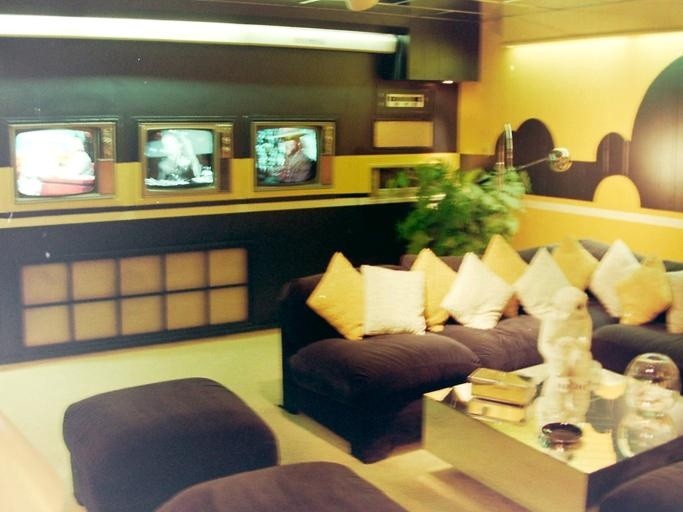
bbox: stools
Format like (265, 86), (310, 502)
(153, 461), (408, 512)
(63, 376), (281, 512)
(600, 460), (683, 512)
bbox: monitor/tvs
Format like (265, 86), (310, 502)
(7, 122), (116, 205)
(251, 121), (336, 192)
(138, 122), (233, 198)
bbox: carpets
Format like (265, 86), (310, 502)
(0, 328), (533, 512)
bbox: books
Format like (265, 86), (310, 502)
(466, 366), (545, 425)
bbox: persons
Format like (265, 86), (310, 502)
(277, 127), (314, 182)
(509, 117), (557, 195)
(151, 132), (202, 181)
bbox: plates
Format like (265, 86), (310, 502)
(540, 421), (584, 443)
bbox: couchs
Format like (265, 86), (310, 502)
(277, 237), (683, 464)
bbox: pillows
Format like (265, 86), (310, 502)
(552, 238), (600, 291)
(514, 248), (575, 323)
(614, 256), (672, 326)
(439, 252), (516, 330)
(481, 234), (530, 318)
(407, 247), (456, 332)
(589, 239), (641, 318)
(305, 252), (363, 342)
(666, 271), (683, 334)
(358, 263), (427, 336)
